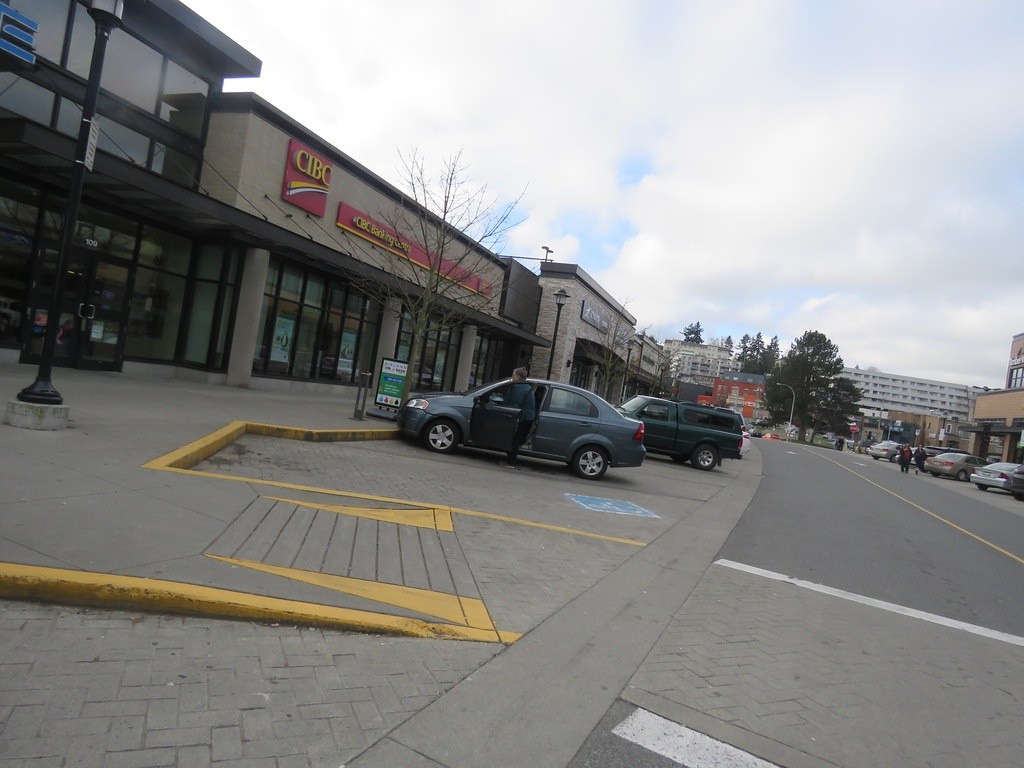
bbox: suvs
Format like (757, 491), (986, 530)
(924, 447), (967, 460)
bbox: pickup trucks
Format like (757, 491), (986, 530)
(613, 395), (744, 470)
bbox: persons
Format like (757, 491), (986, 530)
(913, 445), (926, 475)
(499, 367), (538, 469)
(835, 436), (845, 451)
(897, 443), (913, 474)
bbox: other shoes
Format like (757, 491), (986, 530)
(915, 470), (918, 475)
(498, 460), (516, 469)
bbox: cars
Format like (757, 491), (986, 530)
(868, 441), (905, 463)
(924, 453), (991, 481)
(747, 414), (879, 462)
(714, 406), (750, 459)
(971, 463), (1024, 496)
(397, 377), (647, 481)
(895, 447), (923, 466)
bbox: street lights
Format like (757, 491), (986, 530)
(777, 382), (796, 442)
(17, 0), (124, 404)
(546, 289), (571, 380)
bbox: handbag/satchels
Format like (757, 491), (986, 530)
(914, 457), (916, 461)
(898, 456), (904, 465)
